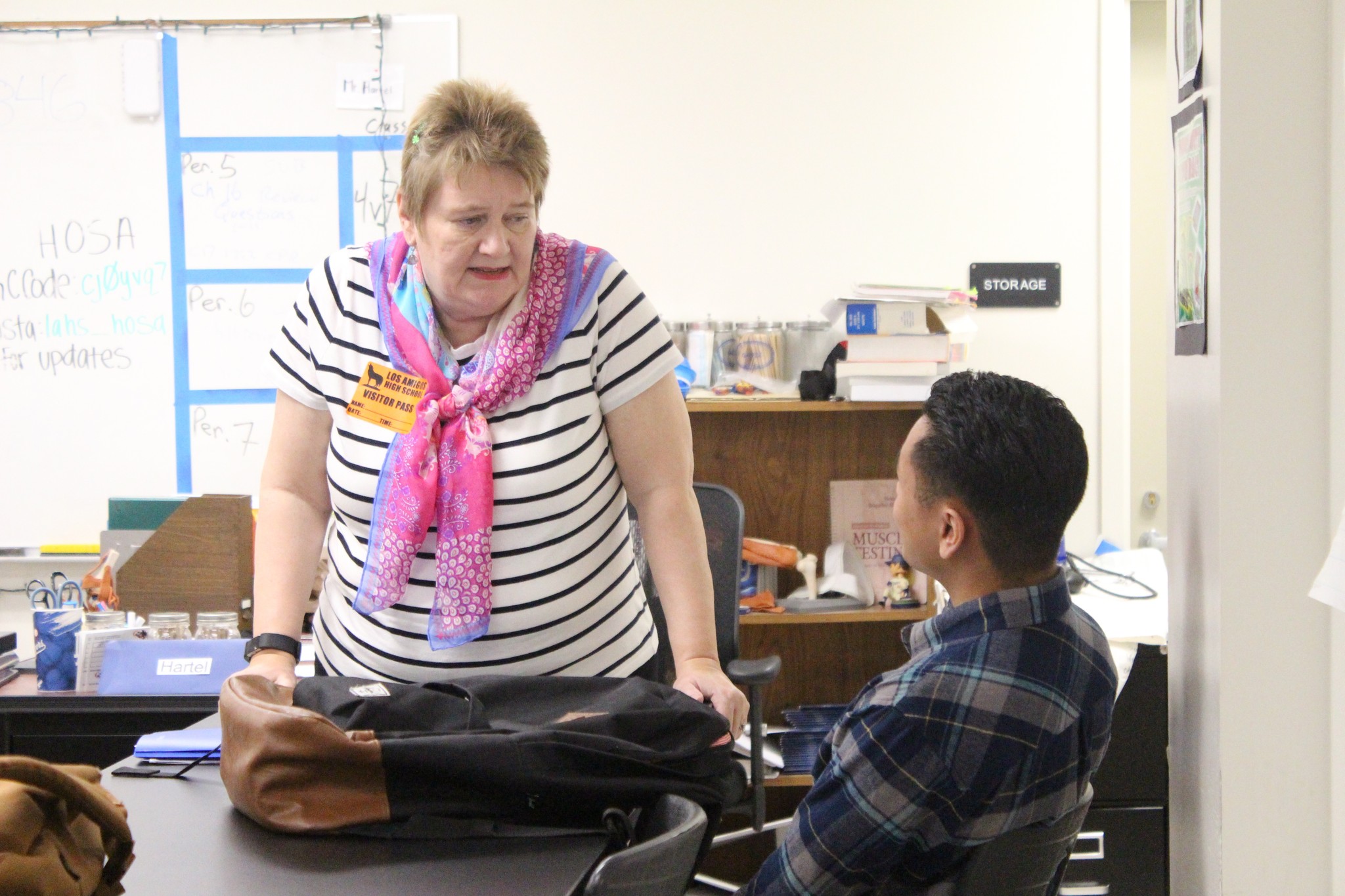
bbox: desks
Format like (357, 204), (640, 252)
(98, 713), (638, 896)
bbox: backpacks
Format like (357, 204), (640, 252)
(215, 670), (751, 836)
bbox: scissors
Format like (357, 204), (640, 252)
(26, 572), (72, 609)
(31, 581), (83, 610)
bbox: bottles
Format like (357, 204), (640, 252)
(662, 320), (831, 391)
(84, 611), (126, 631)
(195, 611), (241, 640)
(149, 613), (193, 641)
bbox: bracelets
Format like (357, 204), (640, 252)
(244, 632), (302, 664)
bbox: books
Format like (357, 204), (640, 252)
(0, 631), (20, 687)
(835, 285), (977, 402)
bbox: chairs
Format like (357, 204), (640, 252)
(957, 782), (1093, 896)
(584, 482), (790, 896)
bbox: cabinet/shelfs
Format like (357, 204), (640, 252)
(689, 402), (937, 624)
(0, 668), (219, 770)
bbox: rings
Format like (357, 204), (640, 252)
(739, 725), (745, 731)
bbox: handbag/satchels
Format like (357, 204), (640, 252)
(0, 759), (136, 895)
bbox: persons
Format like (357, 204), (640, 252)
(881, 555), (912, 603)
(748, 369), (1118, 896)
(246, 81), (751, 743)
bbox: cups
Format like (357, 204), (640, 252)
(32, 607), (85, 692)
